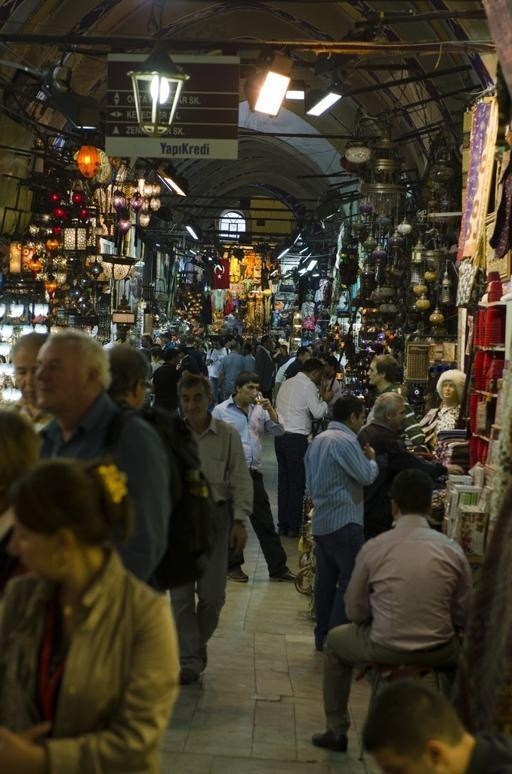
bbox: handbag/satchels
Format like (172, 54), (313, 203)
(140, 407), (218, 592)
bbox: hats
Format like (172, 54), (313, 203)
(435, 369), (468, 406)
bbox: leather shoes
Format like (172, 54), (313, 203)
(311, 731), (349, 753)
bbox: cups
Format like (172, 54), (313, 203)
(255, 393), (264, 405)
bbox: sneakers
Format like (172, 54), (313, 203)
(181, 670), (198, 685)
(314, 633), (331, 651)
(268, 568), (299, 582)
(277, 526), (287, 536)
(226, 565), (248, 581)
(286, 529), (301, 539)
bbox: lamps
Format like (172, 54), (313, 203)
(286, 79), (305, 101)
(244, 70), (289, 115)
(10, 146), (161, 300)
(345, 129), (454, 325)
(112, 295), (134, 343)
(305, 91), (342, 115)
(127, 34), (190, 137)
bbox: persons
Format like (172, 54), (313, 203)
(1, 457), (181, 774)
(303, 394), (379, 652)
(0, 409), (43, 599)
(357, 391), (465, 541)
(362, 676), (512, 773)
(312, 469), (473, 753)
(366, 354), (426, 445)
(103, 343), (202, 594)
(12, 332), (54, 434)
(273, 358), (334, 538)
(169, 374), (255, 684)
(34, 327), (172, 583)
(140, 316), (340, 412)
(418, 369), (467, 450)
(210, 370), (297, 582)
